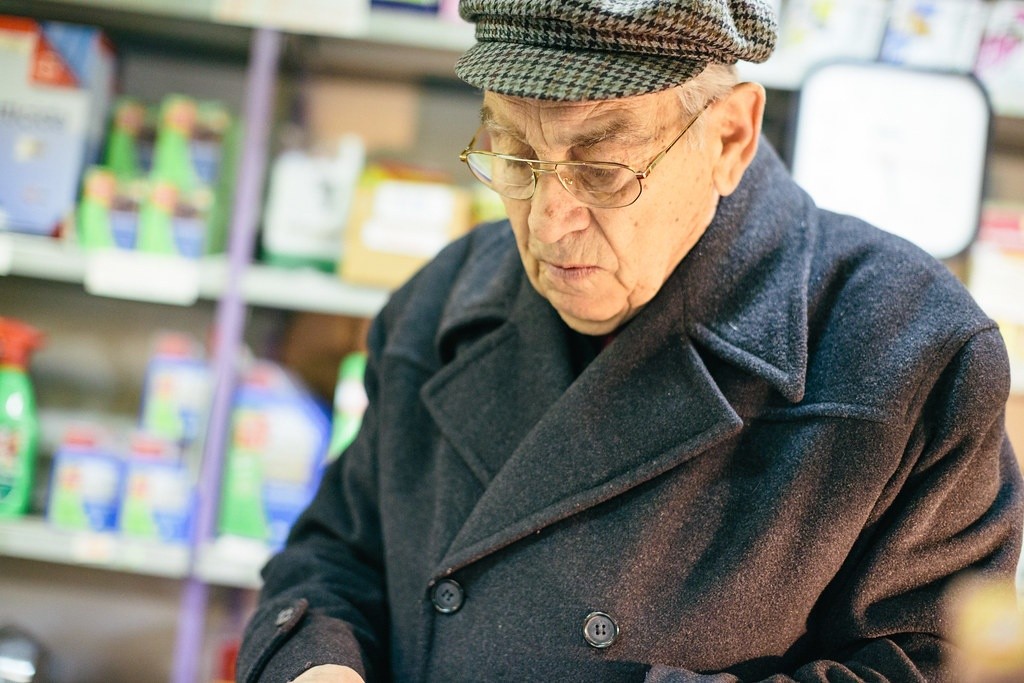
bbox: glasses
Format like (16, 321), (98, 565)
(458, 94), (714, 209)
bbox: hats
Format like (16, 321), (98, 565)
(451, 0), (778, 101)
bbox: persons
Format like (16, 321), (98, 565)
(234, 0), (1024, 683)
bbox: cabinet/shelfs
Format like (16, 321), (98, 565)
(0, 0), (1024, 683)
